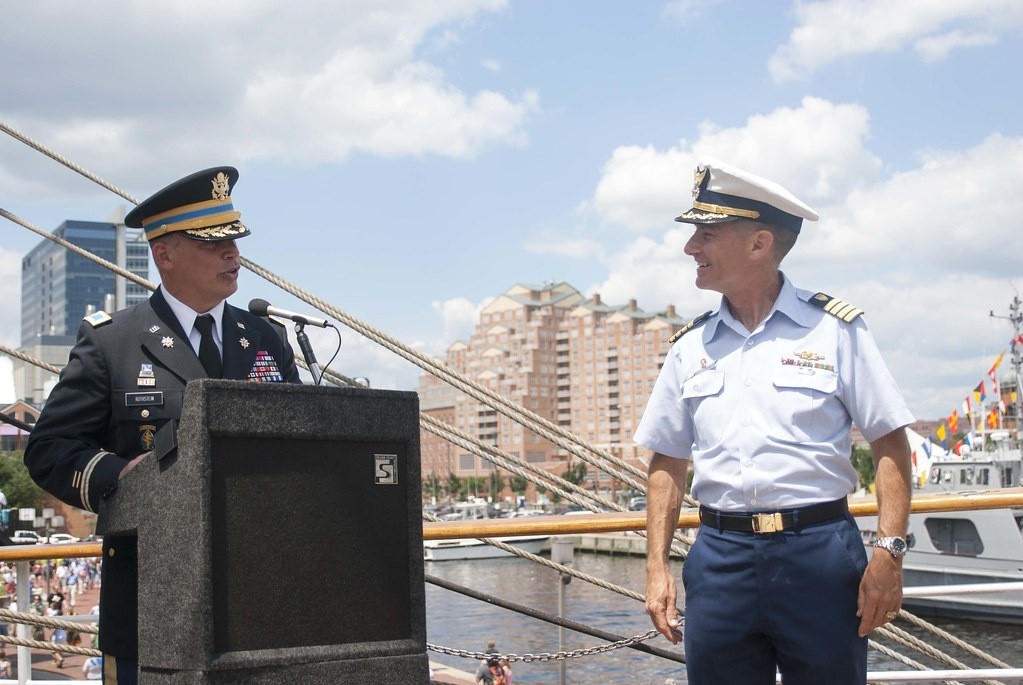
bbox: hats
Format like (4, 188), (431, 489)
(124, 166), (251, 241)
(673, 163), (818, 233)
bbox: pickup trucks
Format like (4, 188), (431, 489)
(8, 530), (47, 544)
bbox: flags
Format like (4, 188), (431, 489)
(975, 383), (985, 402)
(963, 398), (970, 414)
(953, 442), (963, 455)
(987, 331), (1023, 416)
(937, 425), (947, 450)
(922, 436), (932, 459)
(920, 471), (926, 486)
(948, 411), (958, 433)
(988, 410), (998, 428)
(964, 433), (976, 449)
(911, 450), (918, 466)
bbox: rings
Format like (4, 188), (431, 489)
(886, 611), (896, 619)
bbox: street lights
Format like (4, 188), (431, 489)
(550, 537), (575, 685)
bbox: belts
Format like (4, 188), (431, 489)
(700, 498), (850, 534)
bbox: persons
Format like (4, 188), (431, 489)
(475, 641), (512, 685)
(0, 556), (105, 680)
(633, 165), (916, 685)
(23, 166), (302, 685)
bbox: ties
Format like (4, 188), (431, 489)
(193, 315), (225, 381)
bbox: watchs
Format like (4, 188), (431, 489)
(873, 537), (907, 558)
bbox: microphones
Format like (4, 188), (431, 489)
(248, 299), (334, 329)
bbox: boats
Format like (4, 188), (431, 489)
(424, 536), (549, 561)
(846, 298), (1023, 624)
(438, 500), (503, 520)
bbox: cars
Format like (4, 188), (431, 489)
(37, 534), (81, 545)
(629, 498), (646, 511)
